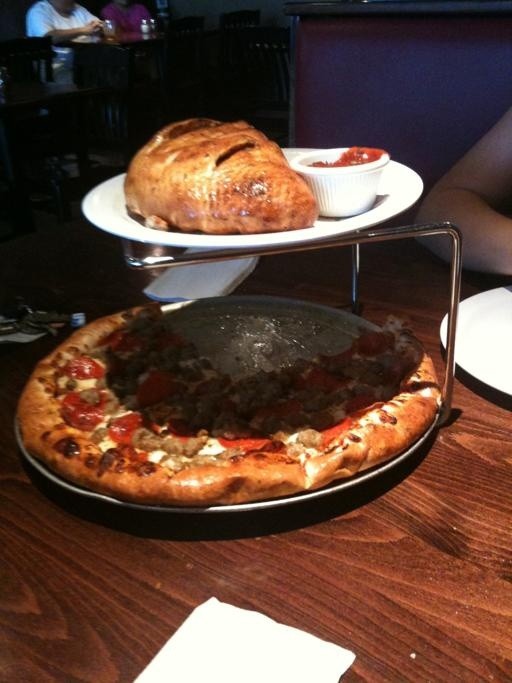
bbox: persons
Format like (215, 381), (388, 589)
(98, 0), (153, 33)
(24, 0), (105, 85)
(411, 104), (511, 281)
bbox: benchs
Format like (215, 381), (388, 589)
(286, 11), (511, 263)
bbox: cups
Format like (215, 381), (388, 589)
(288, 146), (390, 220)
(102, 19), (117, 39)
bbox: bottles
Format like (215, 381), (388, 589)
(140, 16), (155, 34)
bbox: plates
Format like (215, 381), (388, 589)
(438, 283), (512, 397)
(80, 149), (427, 252)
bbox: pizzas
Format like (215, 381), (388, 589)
(16, 301), (442, 507)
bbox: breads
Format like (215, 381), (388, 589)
(124, 116), (320, 235)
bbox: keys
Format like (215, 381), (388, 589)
(0, 301), (68, 340)
